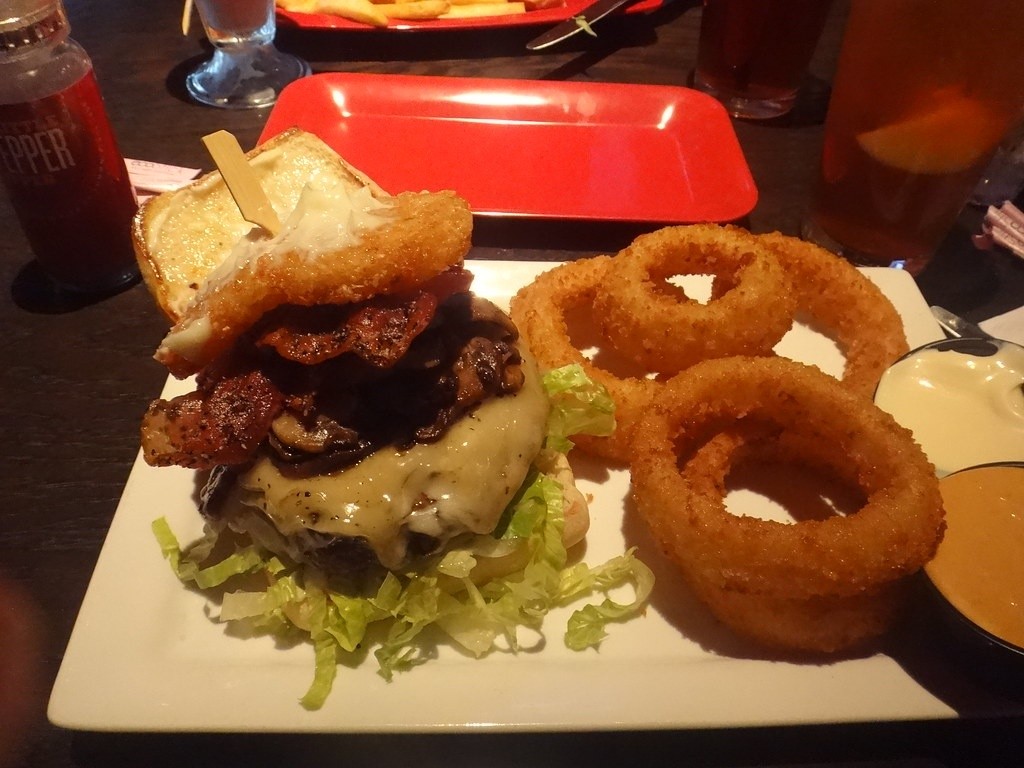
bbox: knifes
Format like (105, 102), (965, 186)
(526, 0), (632, 51)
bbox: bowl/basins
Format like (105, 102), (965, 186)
(865, 336), (1022, 662)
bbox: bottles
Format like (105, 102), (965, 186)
(0, 0), (158, 290)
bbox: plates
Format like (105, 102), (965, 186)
(256, 71), (762, 232)
(43, 259), (1023, 731)
(278, 2), (664, 31)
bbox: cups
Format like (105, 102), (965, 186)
(184, 2), (312, 109)
